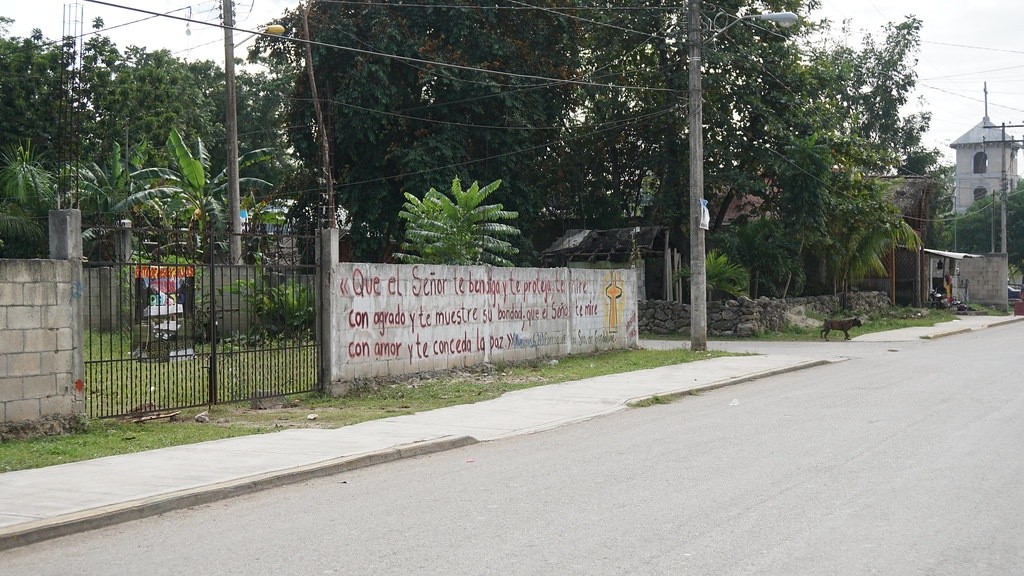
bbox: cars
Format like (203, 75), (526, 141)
(1008, 285), (1022, 299)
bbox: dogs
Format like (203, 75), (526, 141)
(820, 317), (863, 342)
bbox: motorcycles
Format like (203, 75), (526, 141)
(930, 288), (951, 309)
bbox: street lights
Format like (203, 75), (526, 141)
(688, 1), (797, 353)
(224, 1), (291, 265)
